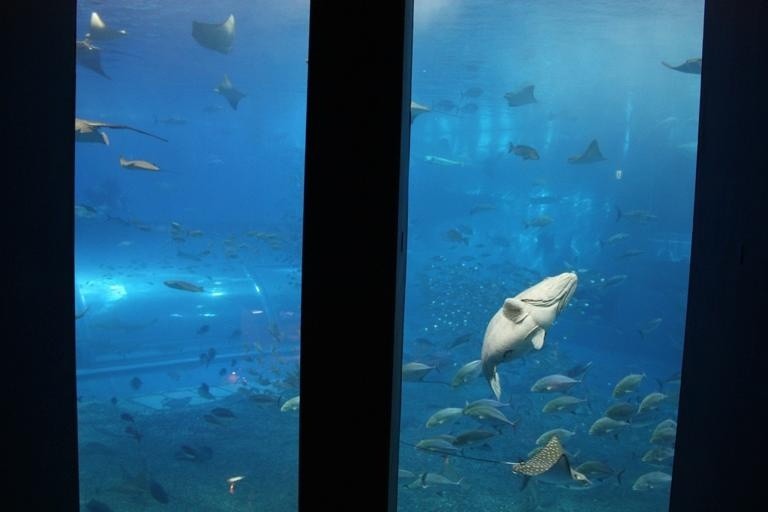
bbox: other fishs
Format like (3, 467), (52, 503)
(400, 197), (677, 496)
(72, 201), (303, 512)
